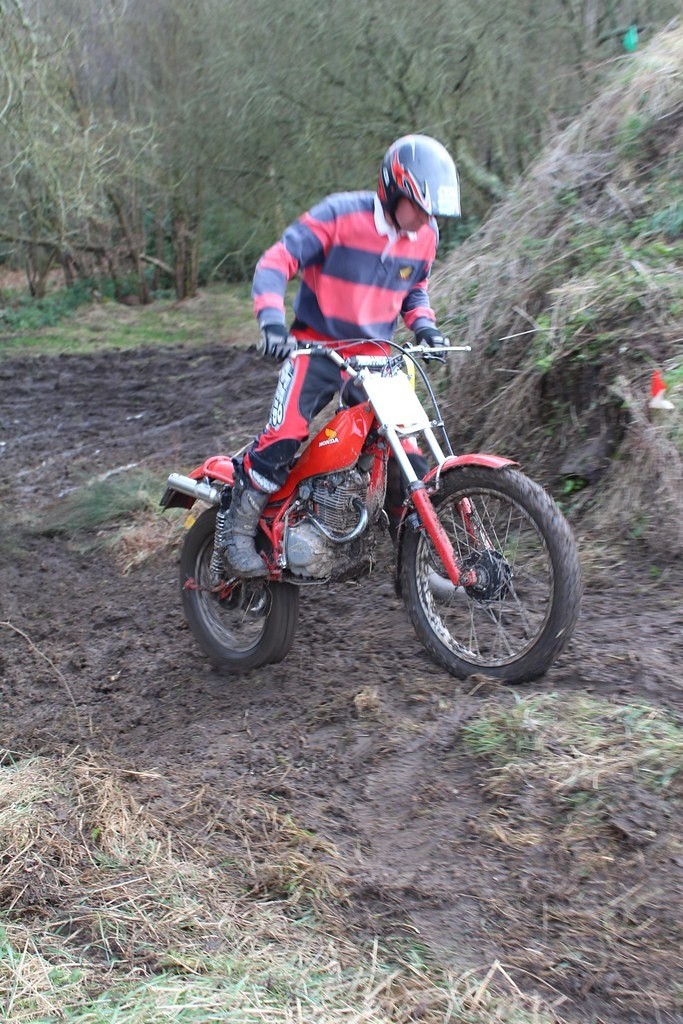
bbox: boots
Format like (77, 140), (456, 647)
(389, 519), (465, 601)
(218, 464), (271, 580)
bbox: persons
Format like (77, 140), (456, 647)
(221, 132), (464, 582)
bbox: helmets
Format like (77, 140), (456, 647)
(378, 134), (462, 216)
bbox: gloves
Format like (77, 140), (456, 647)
(416, 330), (452, 366)
(261, 325), (292, 362)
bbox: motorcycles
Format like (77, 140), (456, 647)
(159, 336), (581, 686)
